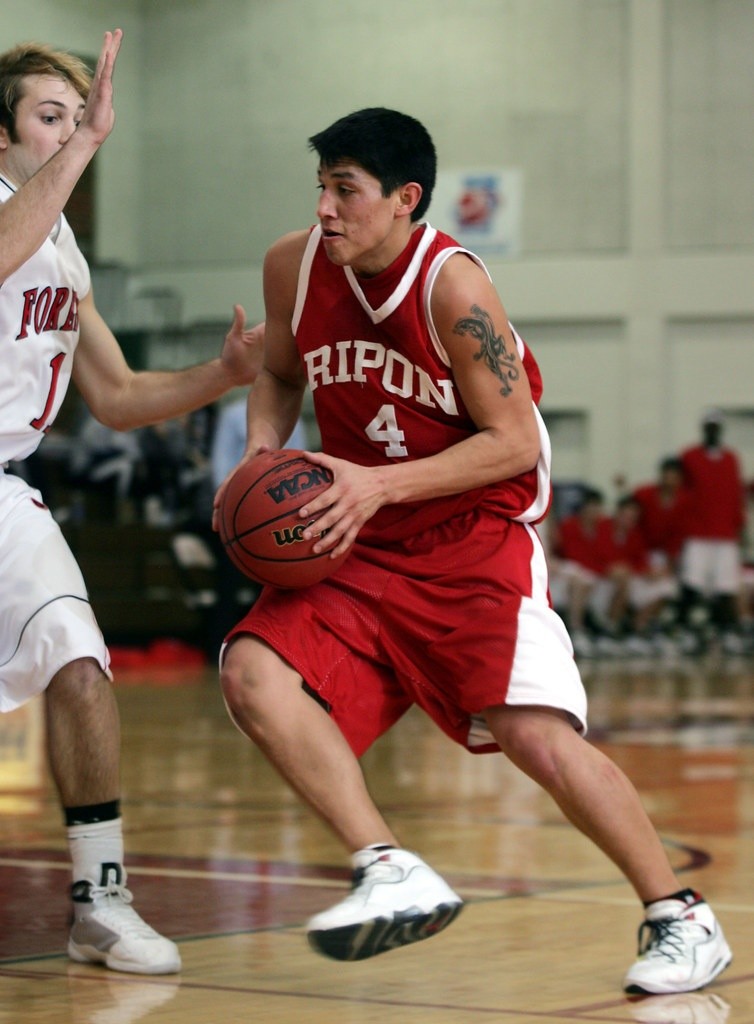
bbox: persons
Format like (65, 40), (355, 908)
(532, 506), (593, 659)
(0, 29), (266, 973)
(209, 397), (307, 669)
(563, 423), (748, 662)
(217, 106), (736, 994)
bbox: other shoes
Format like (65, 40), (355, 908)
(569, 629), (744, 658)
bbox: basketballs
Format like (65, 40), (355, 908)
(213, 447), (357, 595)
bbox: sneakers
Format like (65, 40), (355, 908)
(307, 849), (464, 962)
(622, 898), (733, 1003)
(66, 878), (183, 974)
